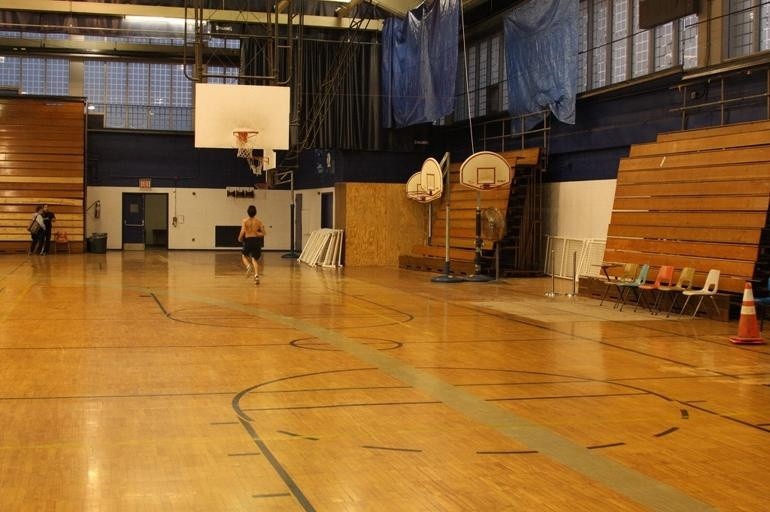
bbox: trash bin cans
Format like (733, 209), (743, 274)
(88, 232), (107, 253)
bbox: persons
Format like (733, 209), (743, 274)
(40, 204), (56, 255)
(28, 206), (46, 255)
(237, 205), (265, 285)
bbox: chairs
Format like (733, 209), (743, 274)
(600, 263), (640, 308)
(54, 231), (70, 255)
(680, 269), (722, 320)
(655, 267), (696, 317)
(592, 263), (626, 305)
(614, 264), (649, 312)
(634, 266), (673, 315)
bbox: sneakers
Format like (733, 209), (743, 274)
(245, 263), (260, 286)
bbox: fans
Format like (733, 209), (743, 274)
(481, 206), (509, 284)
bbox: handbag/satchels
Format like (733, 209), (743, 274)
(27, 216), (40, 235)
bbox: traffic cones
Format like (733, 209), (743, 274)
(728, 281), (770, 345)
